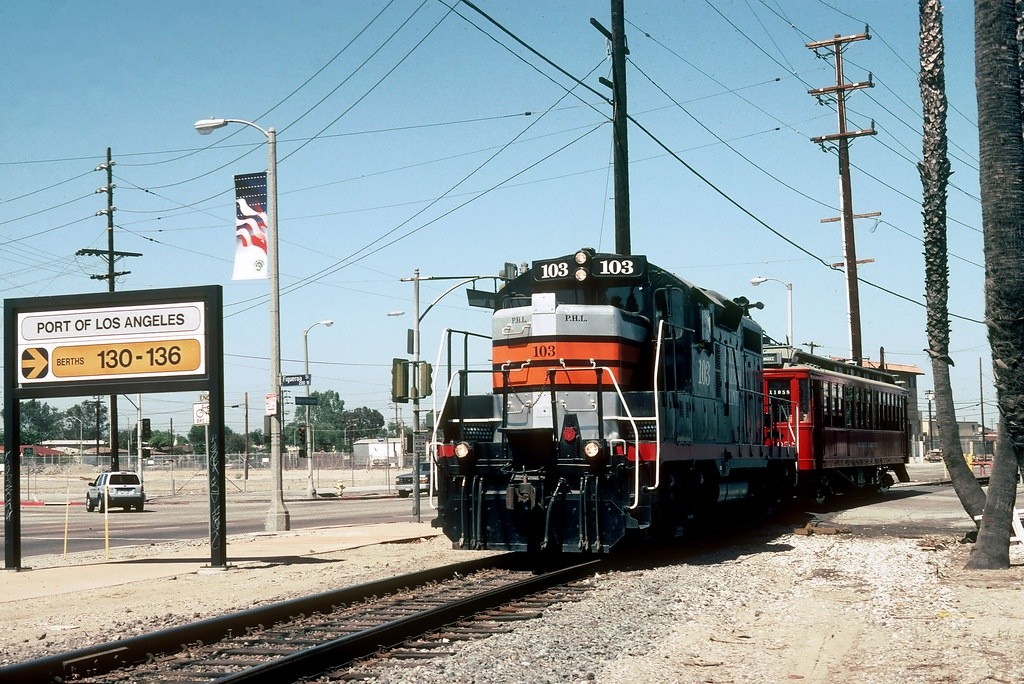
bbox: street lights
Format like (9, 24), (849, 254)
(750, 277), (796, 360)
(304, 320), (334, 500)
(194, 117), (294, 531)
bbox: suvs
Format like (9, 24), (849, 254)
(86, 469), (146, 515)
(395, 462), (439, 499)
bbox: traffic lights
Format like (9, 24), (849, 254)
(299, 428), (306, 446)
(391, 358), (409, 403)
(422, 363), (434, 396)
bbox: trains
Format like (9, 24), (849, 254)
(432, 246), (913, 570)
